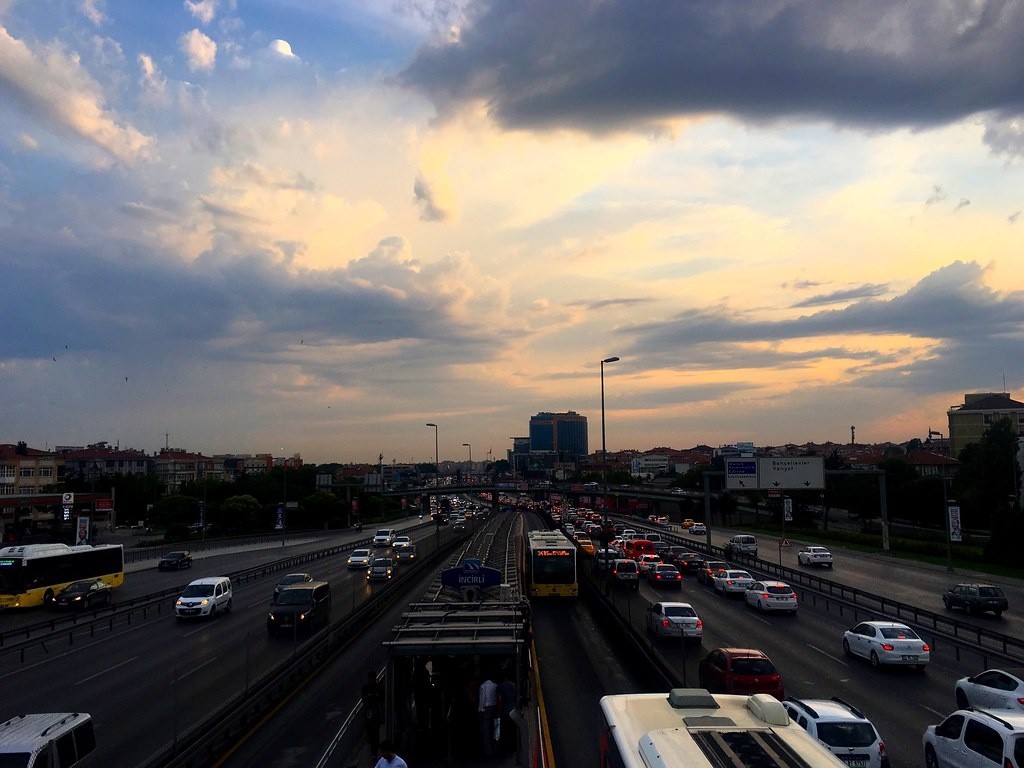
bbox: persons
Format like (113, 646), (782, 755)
(478, 669), (516, 756)
(373, 740), (408, 768)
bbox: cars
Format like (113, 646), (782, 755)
(273, 574), (315, 601)
(797, 545), (834, 569)
(158, 550), (193, 571)
(953, 667), (1024, 711)
(742, 579), (799, 616)
(645, 601), (704, 645)
(843, 620), (931, 673)
(428, 493), (758, 597)
(50, 580), (114, 611)
(697, 647), (786, 702)
(346, 528), (417, 586)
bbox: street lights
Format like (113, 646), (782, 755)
(461, 443), (474, 531)
(600, 356), (621, 555)
(425, 422), (440, 550)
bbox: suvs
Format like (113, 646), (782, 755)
(942, 582), (1009, 618)
(779, 695), (890, 768)
(922, 705), (1024, 768)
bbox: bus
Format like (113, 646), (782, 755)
(526, 528), (580, 601)
(594, 686), (852, 768)
(0, 544), (125, 609)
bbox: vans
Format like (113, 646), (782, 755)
(0, 710), (99, 768)
(267, 581), (332, 642)
(728, 535), (759, 557)
(173, 576), (233, 620)
